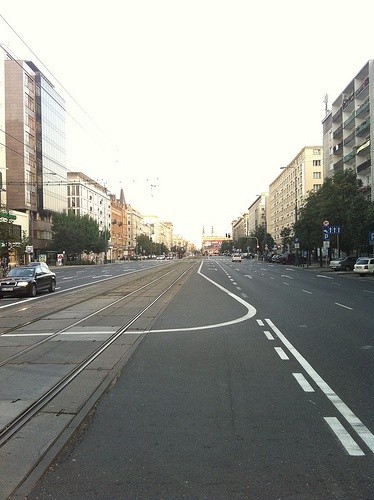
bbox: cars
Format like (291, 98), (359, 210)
(130, 254), (173, 260)
(0, 266), (57, 298)
(329, 256), (359, 271)
(270, 253), (308, 265)
(27, 262), (49, 269)
(232, 253), (242, 262)
(352, 258), (374, 276)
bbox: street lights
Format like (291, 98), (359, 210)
(280, 166), (297, 221)
